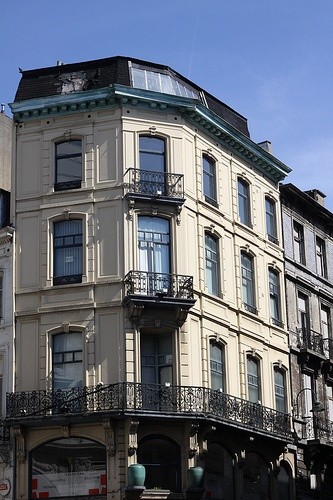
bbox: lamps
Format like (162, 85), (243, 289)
(295, 388), (326, 419)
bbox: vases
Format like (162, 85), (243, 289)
(127, 463), (146, 486)
(187, 467), (205, 490)
